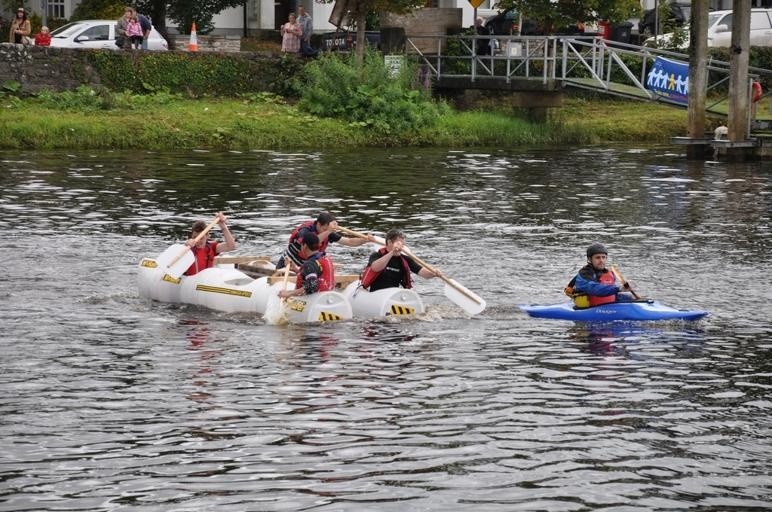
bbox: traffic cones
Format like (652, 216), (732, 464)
(184, 20), (201, 53)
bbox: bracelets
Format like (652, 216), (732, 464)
(221, 227), (226, 231)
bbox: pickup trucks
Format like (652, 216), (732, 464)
(319, 27), (382, 56)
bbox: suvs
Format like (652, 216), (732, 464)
(635, 5), (772, 63)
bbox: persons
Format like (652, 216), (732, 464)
(281, 12), (303, 53)
(564, 243), (633, 309)
(185, 211), (235, 275)
(115, 7), (151, 49)
(8, 7), (31, 44)
(34, 26), (51, 46)
(276, 213), (375, 269)
(277, 234), (335, 298)
(361, 230), (442, 292)
(470, 17), (490, 55)
(296, 4), (313, 50)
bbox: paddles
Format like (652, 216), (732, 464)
(336, 226), (410, 255)
(155, 217), (220, 280)
(264, 262), (289, 324)
(400, 246), (487, 315)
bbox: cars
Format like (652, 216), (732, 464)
(637, 0), (719, 34)
(482, 2), (589, 60)
(27, 14), (171, 53)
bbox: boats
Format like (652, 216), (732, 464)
(516, 291), (709, 323)
(132, 251), (426, 327)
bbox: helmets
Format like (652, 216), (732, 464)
(587, 243), (607, 257)
